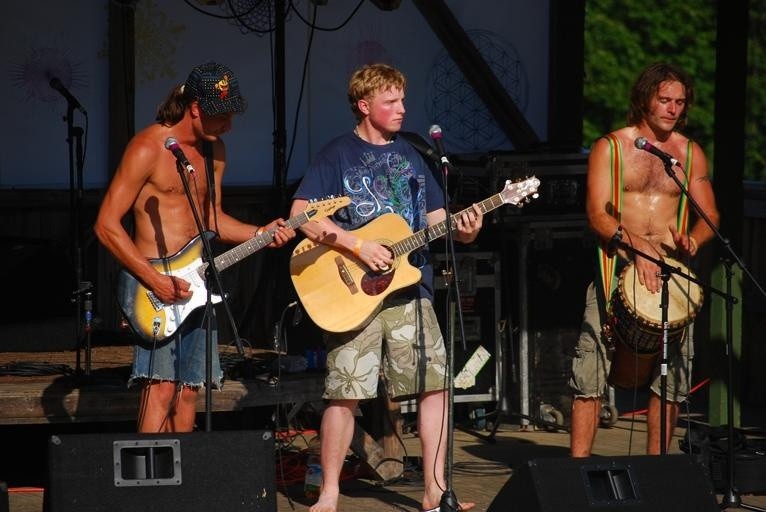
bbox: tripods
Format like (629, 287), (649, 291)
(629, 248), (766, 512)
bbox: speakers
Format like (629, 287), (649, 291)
(44, 429), (276, 512)
(485, 455), (721, 512)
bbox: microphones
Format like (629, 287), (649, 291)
(634, 136), (680, 167)
(165, 136), (194, 175)
(49, 77), (90, 117)
(606, 227), (623, 258)
(430, 124), (450, 168)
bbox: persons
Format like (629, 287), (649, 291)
(93, 61), (297, 433)
(567, 63), (720, 457)
(290, 63), (483, 512)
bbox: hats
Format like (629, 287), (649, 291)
(183, 61), (249, 117)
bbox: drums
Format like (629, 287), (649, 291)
(602, 256), (704, 390)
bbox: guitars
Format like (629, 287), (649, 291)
(289, 175), (541, 333)
(114, 194), (352, 349)
